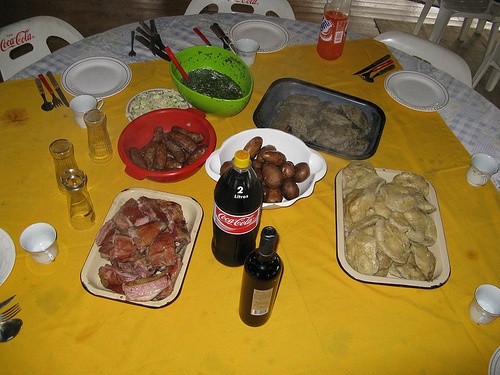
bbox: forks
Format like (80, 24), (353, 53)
(127, 30), (137, 57)
(0, 302), (22, 322)
(38, 74), (63, 109)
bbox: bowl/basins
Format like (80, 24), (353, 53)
(204, 127), (327, 209)
(117, 107), (217, 184)
(169, 45), (254, 118)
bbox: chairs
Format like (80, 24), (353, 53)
(184, 0), (296, 20)
(0, 16), (84, 81)
(373, 31), (473, 88)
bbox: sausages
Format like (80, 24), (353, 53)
(128, 125), (209, 172)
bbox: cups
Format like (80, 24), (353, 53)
(466, 153), (499, 188)
(469, 283), (500, 325)
(228, 38), (260, 68)
(19, 94), (115, 265)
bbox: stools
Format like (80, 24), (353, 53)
(413, 0), (500, 93)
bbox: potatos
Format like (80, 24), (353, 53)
(219, 137), (310, 202)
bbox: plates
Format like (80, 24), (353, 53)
(334, 169), (450, 290)
(61, 20), (449, 160)
(80, 187), (204, 309)
(0, 228), (16, 287)
(487, 346), (500, 375)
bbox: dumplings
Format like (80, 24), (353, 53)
(340, 160), (437, 282)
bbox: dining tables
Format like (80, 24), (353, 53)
(0, 13), (500, 375)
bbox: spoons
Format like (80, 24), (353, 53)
(0, 319), (23, 342)
(366, 64), (396, 83)
(35, 78), (54, 111)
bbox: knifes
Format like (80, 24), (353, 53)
(0, 295), (17, 308)
(135, 35), (171, 62)
(353, 54), (390, 75)
(46, 71), (70, 108)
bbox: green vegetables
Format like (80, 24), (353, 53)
(181, 69), (244, 101)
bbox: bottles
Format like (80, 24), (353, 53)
(237, 225), (284, 330)
(316, 0), (354, 60)
(211, 149), (263, 267)
